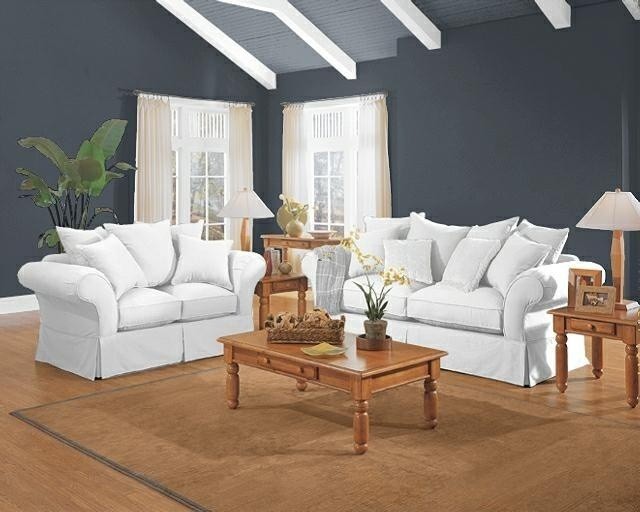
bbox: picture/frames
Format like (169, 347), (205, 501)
(567, 268), (603, 310)
(574, 286), (616, 314)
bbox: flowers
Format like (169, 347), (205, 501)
(284, 203), (308, 219)
(341, 238), (413, 320)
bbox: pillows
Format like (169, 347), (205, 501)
(348, 225), (402, 275)
(516, 219), (572, 266)
(79, 235), (144, 300)
(363, 211), (426, 241)
(466, 215), (520, 284)
(136, 214), (203, 265)
(484, 231), (552, 298)
(441, 239), (502, 293)
(405, 211), (470, 281)
(103, 218), (178, 288)
(55, 226), (104, 269)
(383, 239), (433, 286)
(171, 235), (234, 288)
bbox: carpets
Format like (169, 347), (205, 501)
(7, 363), (623, 512)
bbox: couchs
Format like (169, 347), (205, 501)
(17, 246), (267, 380)
(304, 246), (606, 387)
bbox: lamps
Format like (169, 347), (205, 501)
(216, 188), (274, 250)
(575, 188), (640, 308)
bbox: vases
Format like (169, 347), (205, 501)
(285, 220), (305, 238)
(356, 320), (391, 350)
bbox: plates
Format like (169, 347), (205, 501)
(307, 230), (338, 239)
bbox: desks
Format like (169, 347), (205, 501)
(251, 274), (307, 330)
(546, 307), (640, 407)
(259, 232), (341, 294)
